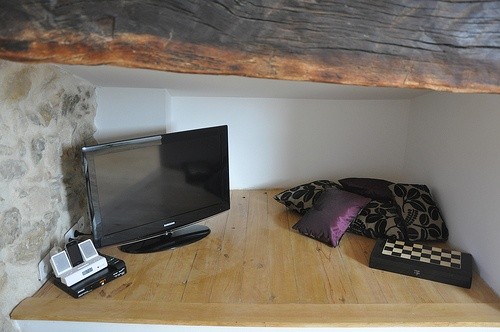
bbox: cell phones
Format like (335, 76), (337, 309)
(65, 241), (83, 267)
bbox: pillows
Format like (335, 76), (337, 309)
(273, 178), (449, 248)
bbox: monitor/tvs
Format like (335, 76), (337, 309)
(83, 125), (230, 253)
